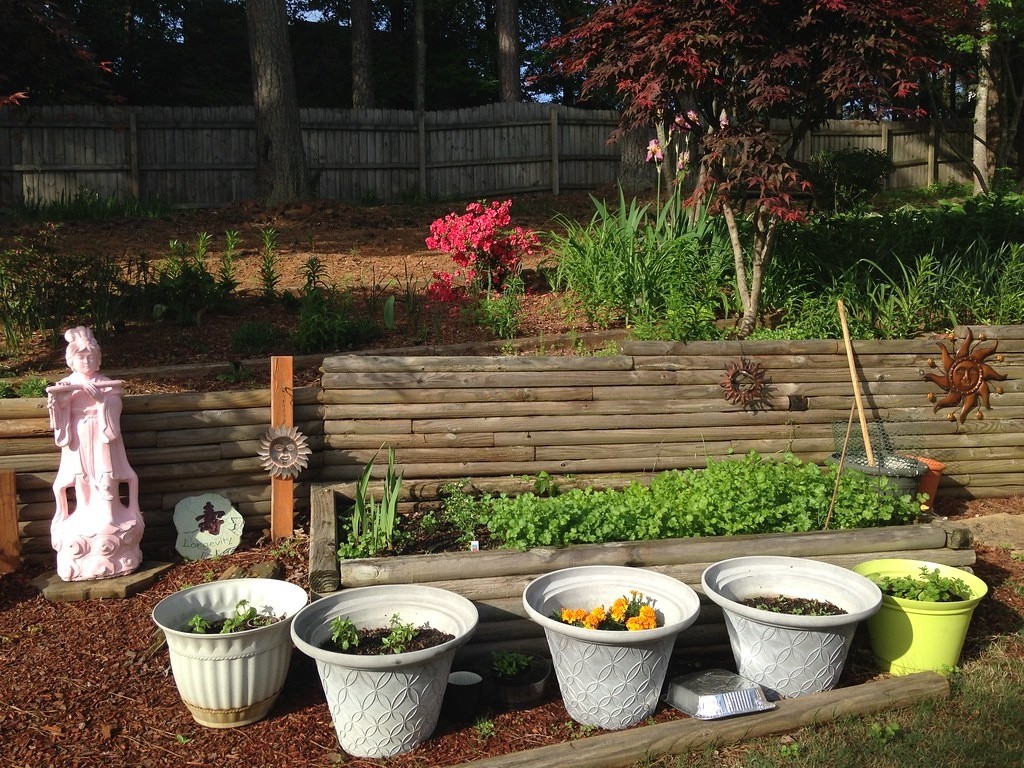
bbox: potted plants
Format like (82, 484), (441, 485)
(852, 559), (988, 676)
(291, 584), (478, 759)
(151, 578), (308, 728)
(701, 555), (883, 702)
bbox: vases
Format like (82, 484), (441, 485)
(522, 565), (700, 731)
(905, 455), (947, 506)
(827, 451), (929, 501)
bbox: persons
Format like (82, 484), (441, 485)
(43, 324), (146, 581)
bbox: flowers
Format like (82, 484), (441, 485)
(547, 591), (662, 631)
